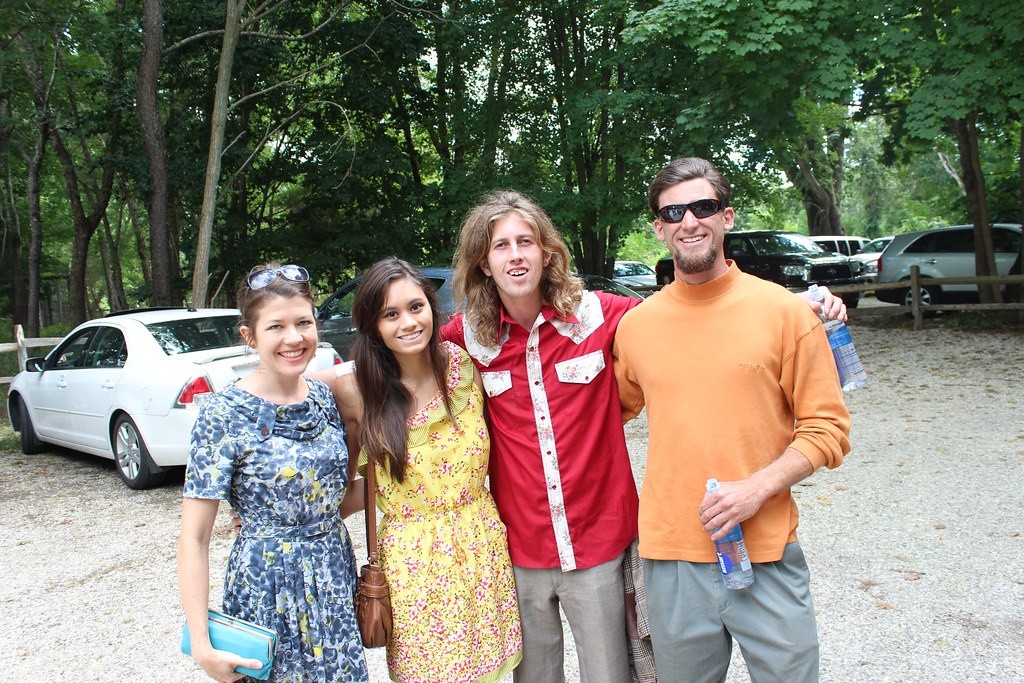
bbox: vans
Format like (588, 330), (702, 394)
(810, 237), (871, 259)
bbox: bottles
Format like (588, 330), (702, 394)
(809, 284), (868, 392)
(702, 478), (754, 590)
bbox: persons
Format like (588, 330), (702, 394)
(303, 191), (847, 683)
(612, 157), (852, 683)
(178, 265), (365, 683)
(333, 258), (523, 683)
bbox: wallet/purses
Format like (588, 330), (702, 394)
(181, 610), (279, 683)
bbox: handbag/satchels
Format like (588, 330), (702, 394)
(350, 562), (394, 649)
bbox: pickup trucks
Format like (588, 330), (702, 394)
(655, 230), (867, 308)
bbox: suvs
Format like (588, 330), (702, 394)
(876, 224), (1024, 320)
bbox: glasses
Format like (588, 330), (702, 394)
(244, 263), (312, 291)
(655, 198), (727, 223)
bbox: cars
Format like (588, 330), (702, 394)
(613, 260), (657, 288)
(847, 236), (895, 282)
(7, 308), (345, 491)
(315, 268), (470, 363)
